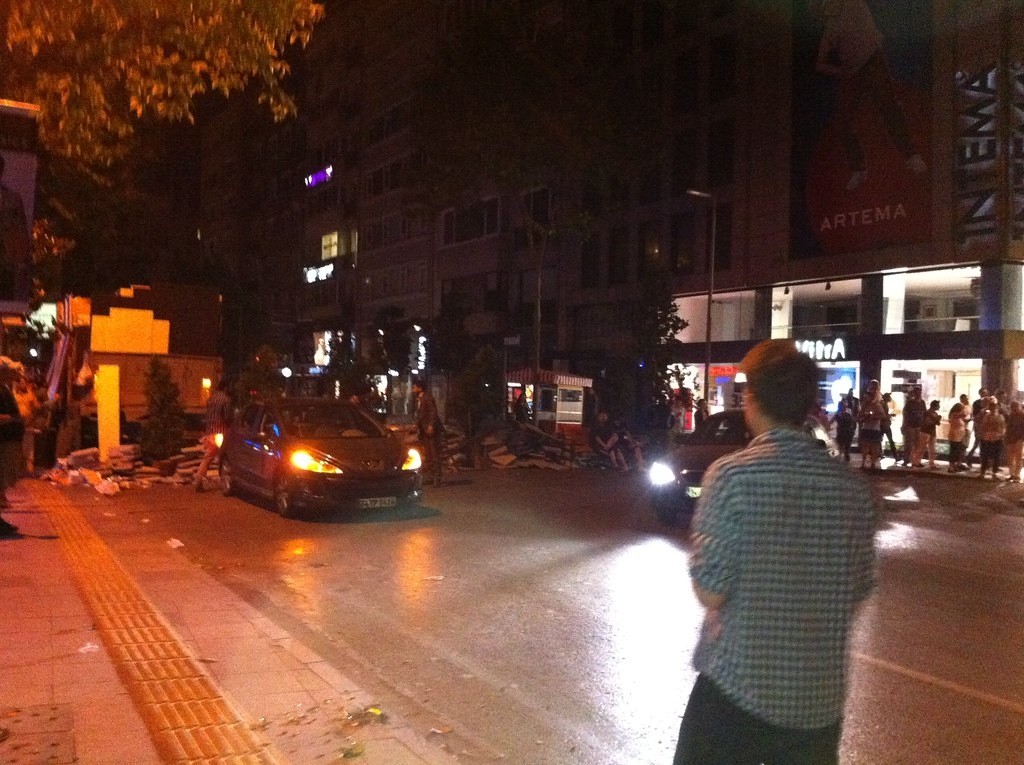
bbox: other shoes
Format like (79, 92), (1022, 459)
(191, 480), (202, 491)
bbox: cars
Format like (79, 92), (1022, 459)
(640, 406), (846, 526)
(215, 397), (426, 519)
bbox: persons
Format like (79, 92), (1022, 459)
(810, 0), (928, 189)
(948, 388), (1024, 479)
(694, 399), (709, 428)
(901, 386), (926, 466)
(911, 399), (942, 468)
(658, 395), (669, 431)
(830, 379), (902, 467)
(0, 157), (29, 300)
(193, 378), (234, 492)
(590, 410), (648, 473)
(672, 337), (876, 765)
(412, 382), (447, 489)
(514, 393), (532, 423)
(0, 356), (41, 539)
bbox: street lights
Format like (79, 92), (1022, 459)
(686, 182), (717, 419)
